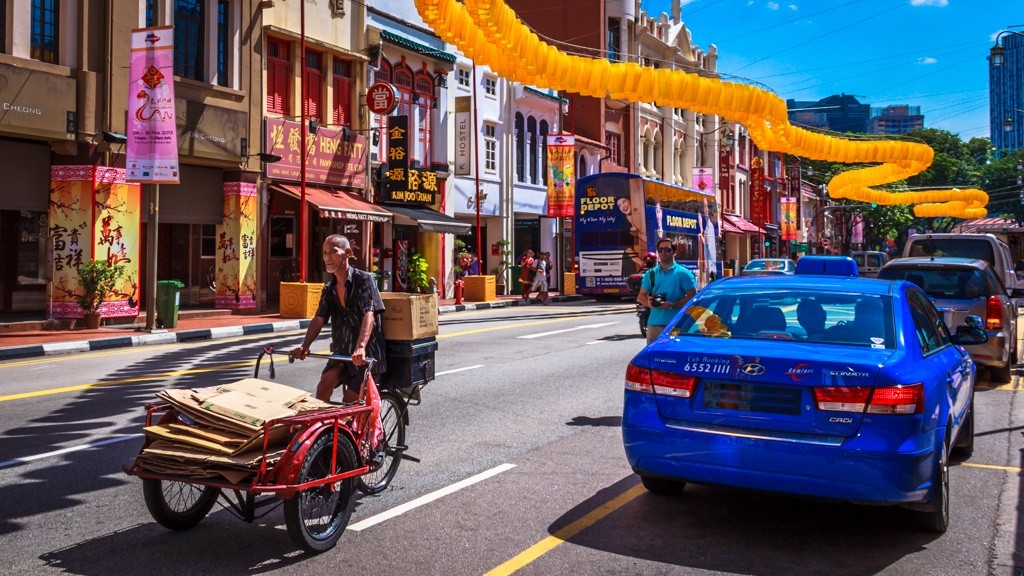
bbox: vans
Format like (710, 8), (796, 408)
(903, 231), (1022, 311)
(847, 251), (891, 278)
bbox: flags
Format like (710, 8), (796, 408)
(850, 213), (863, 243)
(692, 167), (713, 196)
(547, 133), (576, 217)
(125, 26), (181, 185)
(781, 198), (798, 240)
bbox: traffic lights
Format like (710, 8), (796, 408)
(869, 202), (878, 210)
(765, 233), (771, 248)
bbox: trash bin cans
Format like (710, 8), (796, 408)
(156, 279), (185, 329)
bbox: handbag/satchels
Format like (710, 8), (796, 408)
(528, 259), (540, 281)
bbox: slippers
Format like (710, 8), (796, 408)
(366, 444), (389, 472)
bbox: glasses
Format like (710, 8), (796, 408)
(658, 247), (672, 253)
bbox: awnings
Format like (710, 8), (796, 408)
(378, 203), (473, 237)
(960, 217), (1024, 237)
(719, 213), (767, 237)
(270, 184), (392, 224)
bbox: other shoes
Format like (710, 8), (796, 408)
(521, 295), (529, 304)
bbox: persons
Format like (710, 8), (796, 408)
(795, 298), (831, 341)
(637, 238), (696, 349)
(293, 234), (388, 474)
(516, 249), (554, 305)
(617, 198), (632, 228)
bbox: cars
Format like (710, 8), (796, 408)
(876, 253), (1023, 382)
(740, 259), (798, 277)
(622, 256), (991, 530)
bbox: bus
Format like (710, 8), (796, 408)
(572, 171), (727, 303)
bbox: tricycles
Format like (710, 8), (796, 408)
(123, 347), (418, 553)
